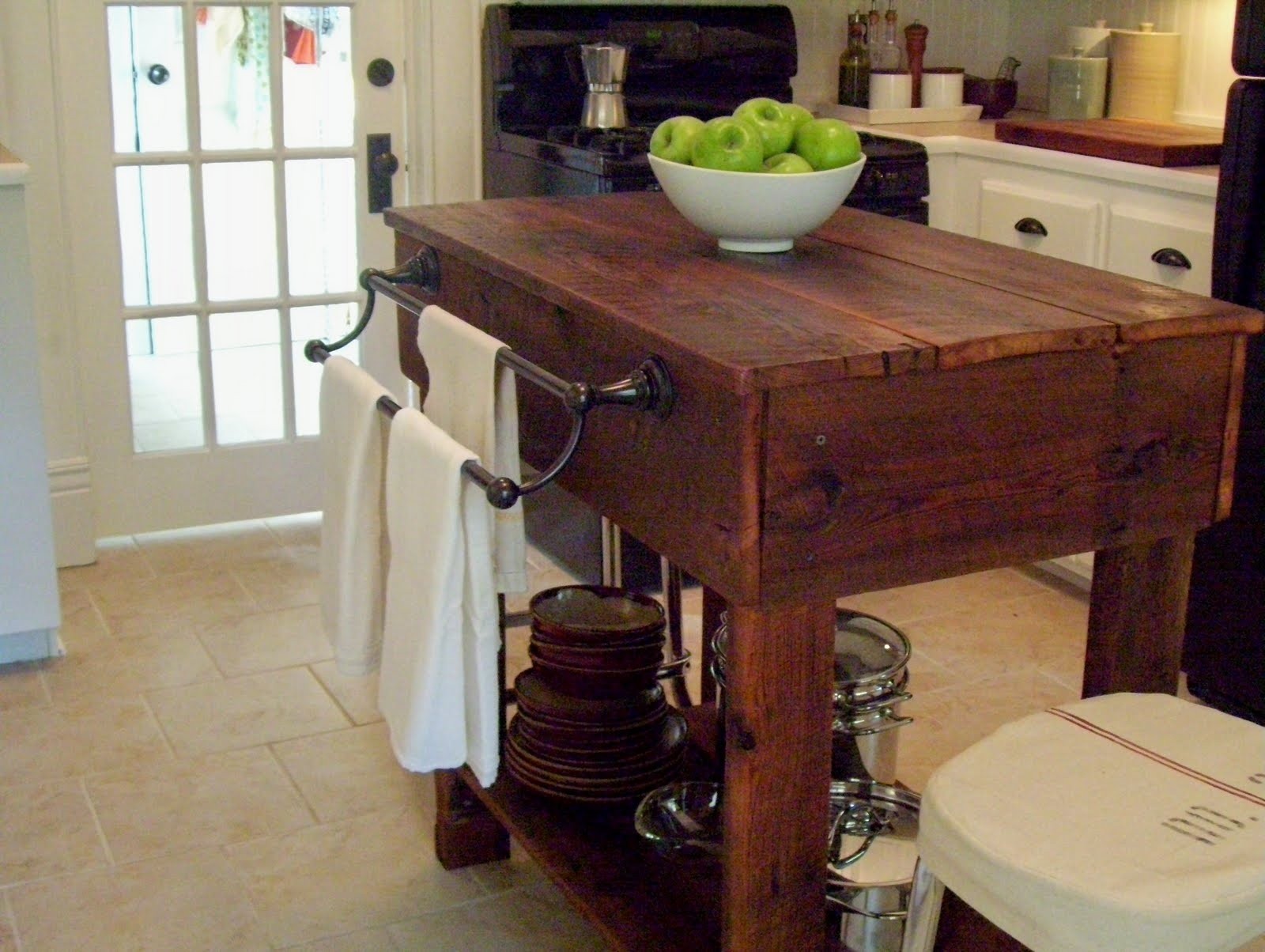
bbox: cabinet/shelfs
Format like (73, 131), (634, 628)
(376, 177), (1265, 952)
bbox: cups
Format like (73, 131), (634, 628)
(1046, 46), (1108, 119)
(1064, 20), (1112, 58)
(868, 71), (912, 110)
(1107, 23), (1182, 123)
(920, 72), (964, 107)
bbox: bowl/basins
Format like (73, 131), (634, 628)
(527, 583), (667, 700)
(645, 151), (867, 253)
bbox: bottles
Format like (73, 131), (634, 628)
(877, 0), (902, 70)
(867, 0), (882, 70)
(839, 10), (870, 108)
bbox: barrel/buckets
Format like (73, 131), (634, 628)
(635, 781), (946, 952)
(709, 608), (911, 786)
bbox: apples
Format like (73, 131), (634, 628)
(757, 153), (813, 173)
(690, 116), (763, 172)
(730, 96), (793, 157)
(781, 103), (813, 150)
(650, 115), (706, 164)
(793, 118), (863, 169)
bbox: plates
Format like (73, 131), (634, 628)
(502, 668), (691, 810)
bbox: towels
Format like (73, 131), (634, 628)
(311, 354), (400, 686)
(415, 302), (533, 594)
(377, 401), (503, 788)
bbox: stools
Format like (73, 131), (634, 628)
(903, 691), (1265, 952)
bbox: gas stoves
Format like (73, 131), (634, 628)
(481, 2), (800, 179)
